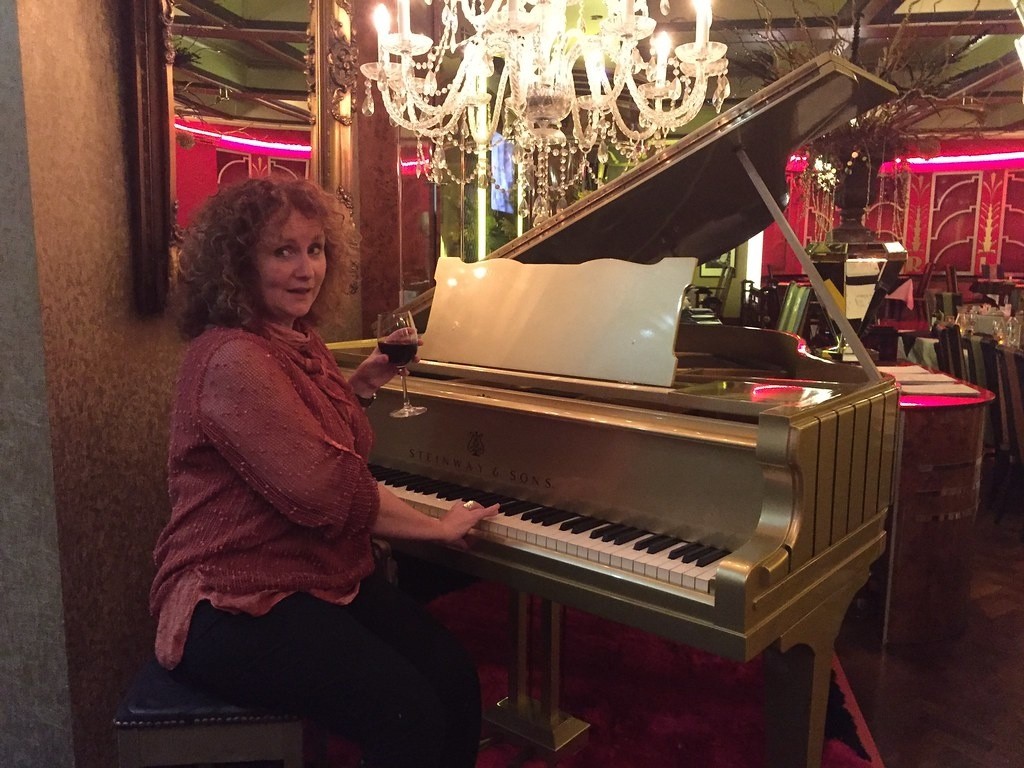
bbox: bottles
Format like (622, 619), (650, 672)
(931, 297), (944, 329)
(1006, 309), (1020, 350)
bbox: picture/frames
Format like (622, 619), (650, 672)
(700, 247), (737, 277)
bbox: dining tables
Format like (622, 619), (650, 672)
(879, 276), (915, 320)
(954, 314), (1024, 351)
(870, 319), (930, 354)
(969, 278), (1022, 308)
(907, 337), (969, 379)
(896, 329), (940, 372)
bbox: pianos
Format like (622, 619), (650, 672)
(325, 54), (902, 768)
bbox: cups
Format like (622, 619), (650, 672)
(944, 316), (955, 323)
(992, 316), (1005, 336)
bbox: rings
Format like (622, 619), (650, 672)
(461, 501), (474, 511)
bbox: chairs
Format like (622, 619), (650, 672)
(934, 322), (969, 382)
(993, 344), (1024, 543)
(686, 266), (736, 324)
(740, 261), (1024, 367)
(965, 334), (1017, 513)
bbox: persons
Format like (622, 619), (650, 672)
(147, 175), (500, 768)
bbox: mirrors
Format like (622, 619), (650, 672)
(128, 1), (361, 319)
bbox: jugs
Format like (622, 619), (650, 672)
(955, 305), (969, 334)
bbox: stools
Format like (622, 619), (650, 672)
(113, 662), (330, 768)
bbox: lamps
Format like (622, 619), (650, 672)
(361, 0), (732, 232)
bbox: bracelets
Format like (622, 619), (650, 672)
(354, 393), (377, 408)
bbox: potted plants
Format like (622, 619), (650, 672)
(712, 1), (1017, 255)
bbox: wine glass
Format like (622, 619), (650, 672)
(376, 309), (427, 418)
